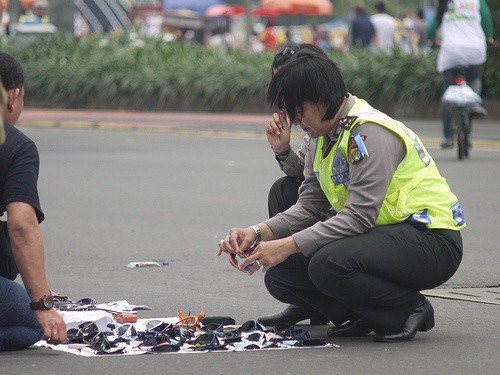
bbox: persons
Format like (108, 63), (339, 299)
(257, 43), (329, 327)
(209, 3), (427, 50)
(0, 52), (67, 351)
(427, 0), (493, 147)
(218, 51), (466, 342)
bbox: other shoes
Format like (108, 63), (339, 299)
(473, 106), (488, 116)
(442, 139), (452, 146)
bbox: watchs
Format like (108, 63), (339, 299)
(30, 295), (53, 310)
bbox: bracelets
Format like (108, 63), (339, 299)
(249, 227), (261, 249)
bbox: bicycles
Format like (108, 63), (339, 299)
(429, 38), (498, 162)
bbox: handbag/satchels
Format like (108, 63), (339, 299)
(441, 80), (482, 105)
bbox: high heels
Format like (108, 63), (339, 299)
(328, 315), (376, 337)
(258, 303), (330, 326)
(374, 297), (435, 342)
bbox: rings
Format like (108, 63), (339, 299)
(51, 332), (58, 335)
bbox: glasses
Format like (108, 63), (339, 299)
(282, 46), (296, 56)
(230, 249), (261, 273)
(48, 296), (328, 355)
